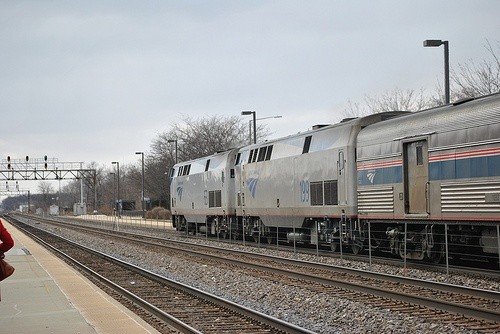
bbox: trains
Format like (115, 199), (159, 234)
(168, 92), (499, 270)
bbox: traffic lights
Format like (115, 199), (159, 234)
(45, 163), (48, 169)
(26, 156), (28, 161)
(45, 156), (48, 161)
(8, 156), (10, 161)
(8, 164), (10, 169)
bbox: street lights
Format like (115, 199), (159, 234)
(168, 139), (177, 164)
(136, 152), (145, 218)
(112, 162), (120, 218)
(241, 111), (256, 144)
(110, 173), (115, 207)
(423, 40), (450, 104)
(249, 116), (282, 143)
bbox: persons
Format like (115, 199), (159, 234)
(0, 220), (14, 302)
